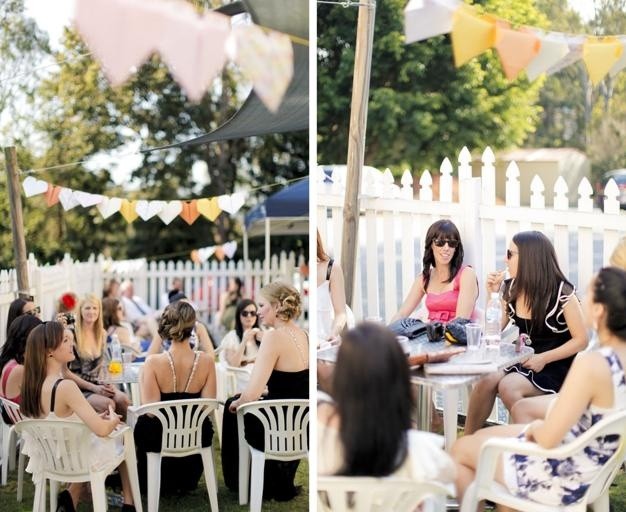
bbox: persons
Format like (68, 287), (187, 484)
(317, 227), (346, 351)
(317, 326), (453, 512)
(445, 267), (625, 510)
(388, 218), (479, 341)
(0, 276), (309, 512)
(463, 230), (590, 432)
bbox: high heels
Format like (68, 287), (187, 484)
(57, 490), (76, 512)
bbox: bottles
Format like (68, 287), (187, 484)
(485, 292), (504, 351)
(109, 334), (121, 361)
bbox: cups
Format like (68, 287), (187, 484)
(366, 316), (383, 323)
(398, 335), (410, 356)
(465, 323), (483, 353)
(108, 360), (123, 377)
(122, 352), (132, 368)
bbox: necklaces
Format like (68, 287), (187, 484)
(520, 290), (537, 345)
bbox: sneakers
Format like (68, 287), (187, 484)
(121, 504), (136, 512)
(432, 237), (459, 248)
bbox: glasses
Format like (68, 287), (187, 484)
(23, 305), (41, 317)
(241, 310), (257, 317)
(507, 249), (522, 260)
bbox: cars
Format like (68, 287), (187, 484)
(593, 165), (626, 212)
